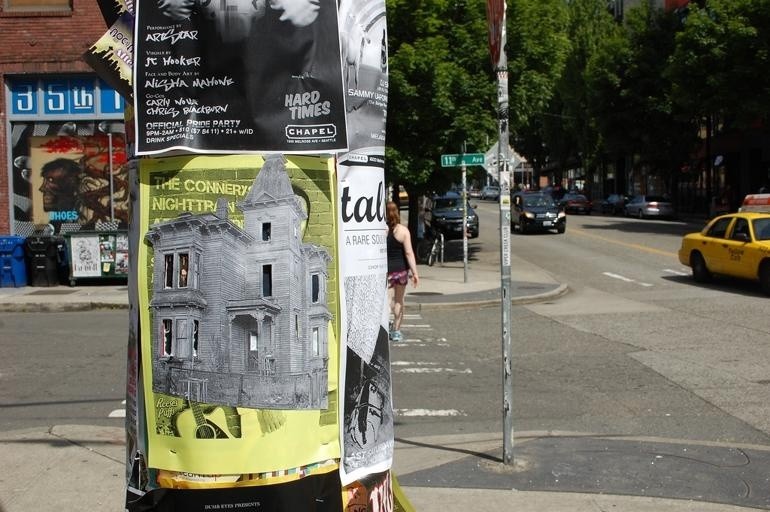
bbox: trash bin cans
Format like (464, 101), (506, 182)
(0, 234), (65, 288)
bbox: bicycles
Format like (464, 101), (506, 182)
(417, 229), (442, 266)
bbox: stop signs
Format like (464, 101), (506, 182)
(486, 0), (505, 65)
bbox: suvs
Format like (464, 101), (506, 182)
(424, 194), (478, 240)
(511, 192), (566, 235)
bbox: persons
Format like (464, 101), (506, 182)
(512, 180), (588, 197)
(386, 202), (419, 341)
(38, 155), (82, 231)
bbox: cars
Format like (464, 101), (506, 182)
(679, 211), (769, 295)
(458, 186), (500, 200)
(541, 186), (593, 215)
(603, 194), (674, 218)
(388, 184), (408, 208)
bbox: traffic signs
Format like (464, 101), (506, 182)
(441, 153), (484, 167)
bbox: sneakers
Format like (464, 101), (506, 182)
(389, 321), (404, 341)
